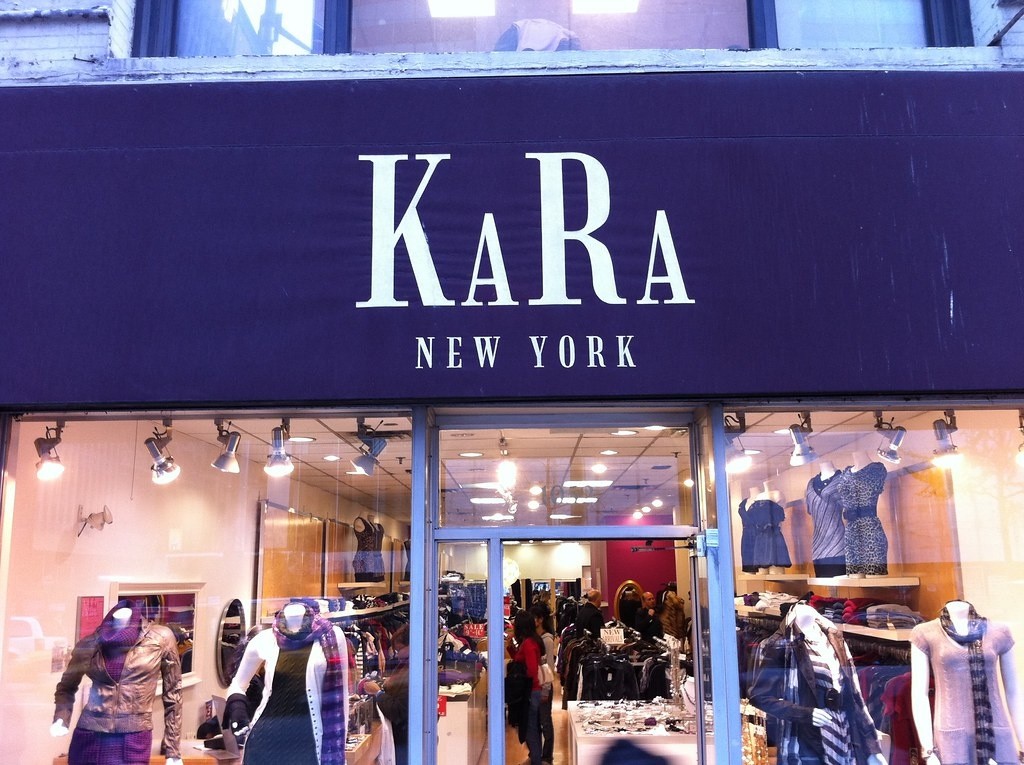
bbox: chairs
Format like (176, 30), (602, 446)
(738, 616), (912, 665)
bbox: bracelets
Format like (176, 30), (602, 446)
(1018, 750), (1024, 763)
(375, 690), (383, 702)
(921, 747), (939, 761)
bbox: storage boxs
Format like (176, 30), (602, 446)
(344, 733), (372, 764)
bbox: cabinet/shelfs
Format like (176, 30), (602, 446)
(438, 667), (486, 765)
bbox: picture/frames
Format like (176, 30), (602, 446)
(111, 581), (205, 696)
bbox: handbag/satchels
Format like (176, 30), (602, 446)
(463, 623), (485, 638)
(537, 663), (556, 686)
(376, 704), (395, 765)
(438, 637), (485, 698)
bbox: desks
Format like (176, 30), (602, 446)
(344, 720), (383, 765)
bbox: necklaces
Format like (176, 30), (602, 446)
(577, 647), (706, 736)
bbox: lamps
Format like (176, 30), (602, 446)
(211, 418), (242, 474)
(931, 409), (958, 454)
(145, 418), (182, 484)
(871, 410), (906, 464)
(264, 417), (294, 477)
(788, 410), (818, 468)
(33, 421), (69, 481)
(77, 503), (114, 531)
(1018, 409), (1024, 451)
(349, 418), (387, 477)
(723, 412), (753, 473)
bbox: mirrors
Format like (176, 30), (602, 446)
(217, 598), (246, 686)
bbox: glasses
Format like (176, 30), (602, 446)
(395, 646), (407, 654)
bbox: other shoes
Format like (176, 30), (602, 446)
(541, 760), (554, 765)
(518, 758), (531, 765)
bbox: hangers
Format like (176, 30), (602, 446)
(567, 616), (685, 671)
(333, 605), (415, 633)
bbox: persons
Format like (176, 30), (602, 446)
(505, 590), (558, 764)
(363, 623), (440, 765)
(221, 601), (350, 765)
(803, 460), (846, 578)
(738, 480), (792, 577)
(663, 591), (687, 653)
(576, 588), (606, 637)
(49, 600), (183, 765)
(747, 603), (887, 765)
(634, 591), (664, 640)
(352, 511), (386, 583)
(836, 449), (888, 578)
(909, 599), (1024, 765)
